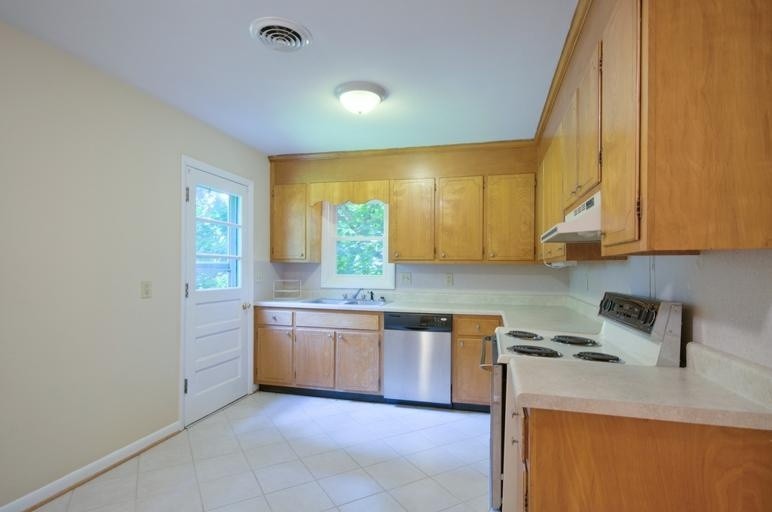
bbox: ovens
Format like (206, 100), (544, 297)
(481, 332), (507, 510)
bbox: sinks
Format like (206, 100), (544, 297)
(344, 299), (395, 305)
(300, 297), (350, 305)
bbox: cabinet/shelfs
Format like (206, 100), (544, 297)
(558, 40), (602, 214)
(290, 310), (384, 399)
(389, 176), (436, 266)
(603, 2), (772, 262)
(250, 308), (291, 392)
(536, 127), (626, 261)
(483, 173), (536, 266)
(270, 184), (319, 264)
(502, 372), (770, 511)
(453, 315), (501, 414)
(437, 178), (483, 266)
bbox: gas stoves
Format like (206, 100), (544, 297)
(493, 324), (679, 372)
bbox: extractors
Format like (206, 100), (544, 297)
(541, 194), (600, 242)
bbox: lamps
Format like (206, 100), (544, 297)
(332, 81), (388, 116)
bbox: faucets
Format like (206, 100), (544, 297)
(370, 291), (374, 301)
(351, 288), (363, 299)
